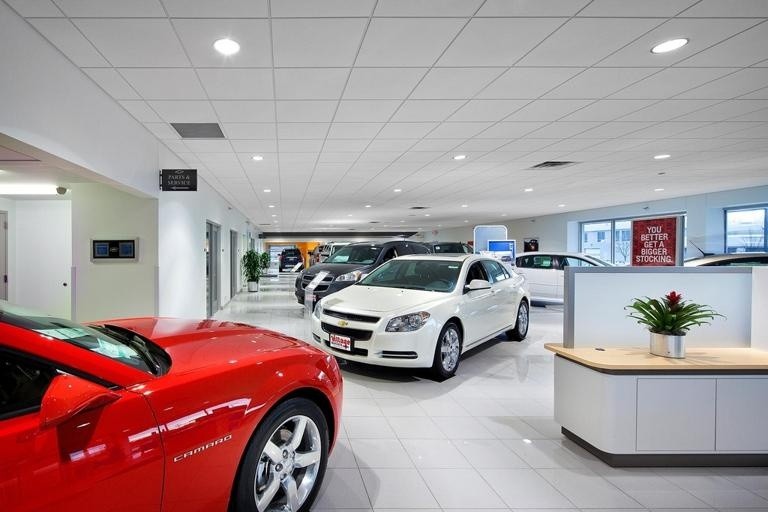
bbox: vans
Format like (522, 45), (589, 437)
(305, 242), (347, 267)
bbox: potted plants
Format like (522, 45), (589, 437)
(240, 249), (271, 292)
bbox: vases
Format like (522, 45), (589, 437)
(649, 331), (686, 359)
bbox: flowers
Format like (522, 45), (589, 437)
(623, 289), (726, 334)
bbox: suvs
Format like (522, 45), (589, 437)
(278, 248), (304, 272)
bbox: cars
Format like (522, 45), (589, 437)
(683, 242), (768, 266)
(0, 301), (345, 512)
(294, 240), (434, 311)
(310, 252), (530, 381)
(424, 242), (474, 254)
(509, 252), (609, 305)
